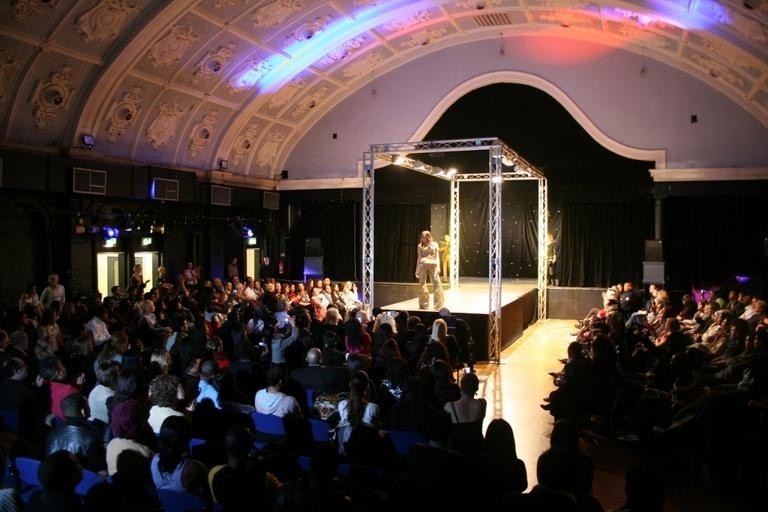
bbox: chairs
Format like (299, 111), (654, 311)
(0, 289), (768, 512)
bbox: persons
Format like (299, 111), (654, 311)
(1, 275), (529, 511)
(229, 256), (238, 275)
(259, 256), (274, 277)
(415, 230), (444, 311)
(530, 282), (768, 511)
(132, 263), (149, 293)
(155, 266), (166, 284)
(440, 234), (450, 281)
(547, 233), (560, 286)
(183, 261), (199, 287)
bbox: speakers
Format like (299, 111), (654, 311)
(305, 238), (321, 256)
(644, 241), (664, 262)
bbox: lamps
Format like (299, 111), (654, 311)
(280, 170), (288, 179)
(81, 134), (96, 151)
(370, 70), (377, 98)
(639, 47), (647, 76)
(218, 160), (228, 171)
(498, 32), (505, 58)
